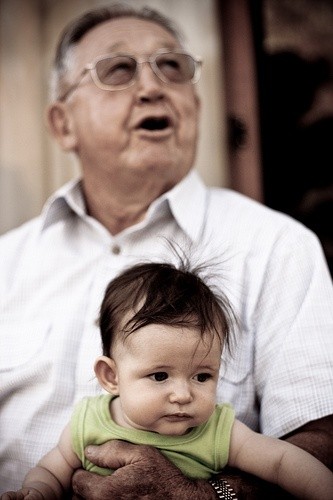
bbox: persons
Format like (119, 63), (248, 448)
(0, 5), (333, 500)
(0, 261), (333, 500)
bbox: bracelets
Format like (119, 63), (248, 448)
(207, 477), (238, 500)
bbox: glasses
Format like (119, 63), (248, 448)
(57, 49), (202, 104)
